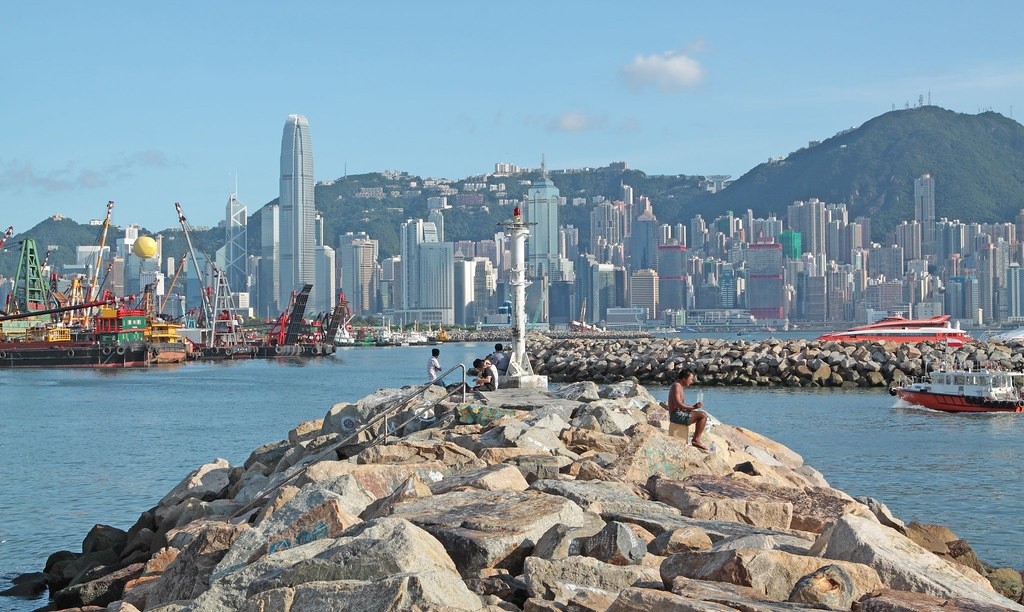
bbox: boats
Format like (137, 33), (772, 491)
(0, 196), (349, 369)
(814, 309), (975, 345)
(887, 333), (1024, 413)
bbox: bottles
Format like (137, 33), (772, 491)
(696, 389), (703, 411)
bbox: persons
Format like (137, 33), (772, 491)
(472, 358), (500, 392)
(426, 348), (446, 388)
(668, 368), (712, 450)
(487, 343), (511, 376)
(939, 359), (1002, 374)
